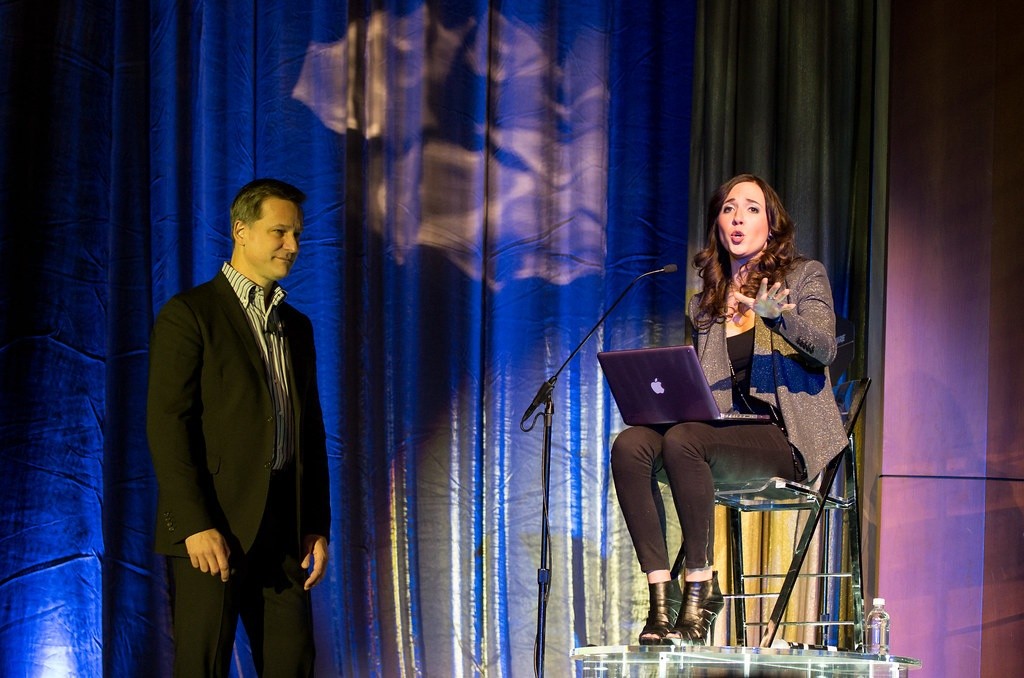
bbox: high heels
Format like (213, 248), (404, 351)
(662, 571), (724, 646)
(638, 572), (683, 645)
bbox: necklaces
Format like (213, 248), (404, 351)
(730, 282), (740, 304)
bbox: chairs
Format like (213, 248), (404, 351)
(639, 377), (870, 650)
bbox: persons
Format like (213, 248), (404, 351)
(145, 179), (332, 678)
(611, 173), (849, 645)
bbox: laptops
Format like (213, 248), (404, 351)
(596, 345), (770, 426)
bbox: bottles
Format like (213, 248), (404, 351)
(865, 598), (890, 655)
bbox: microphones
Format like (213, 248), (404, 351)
(522, 263), (678, 423)
